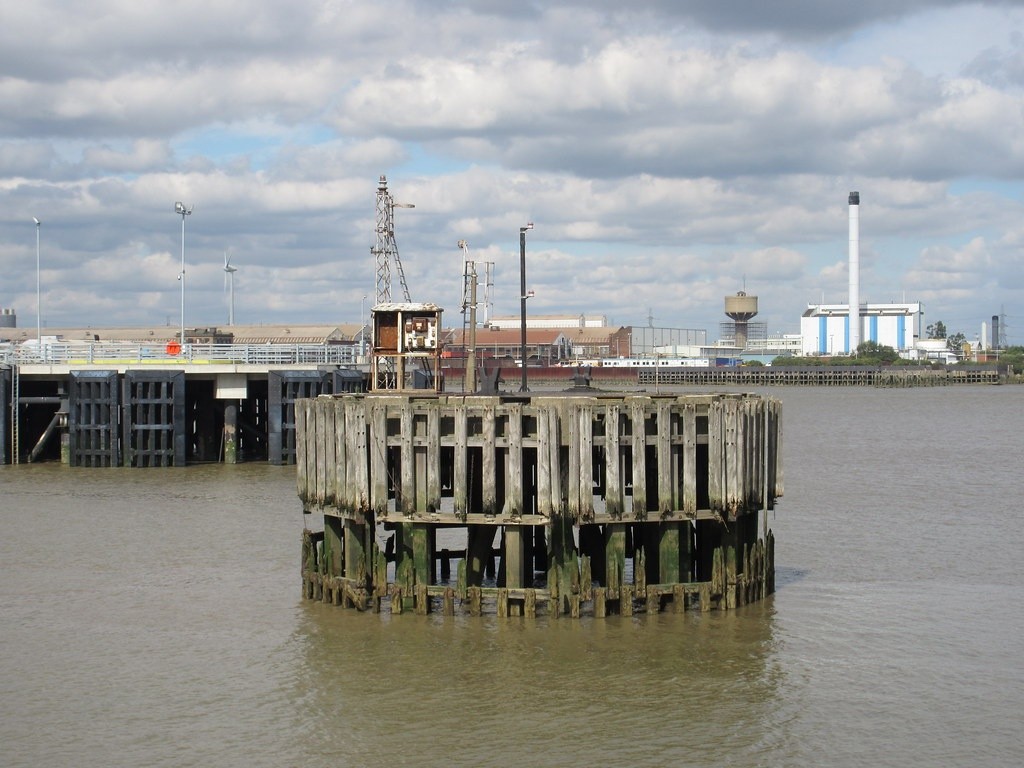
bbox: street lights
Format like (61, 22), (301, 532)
(627, 332), (631, 359)
(32, 216), (41, 358)
(361, 294), (369, 341)
(173, 201), (196, 355)
